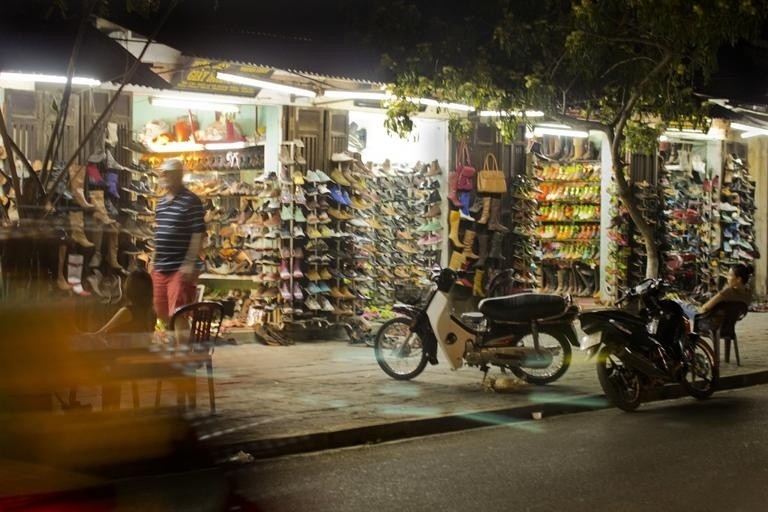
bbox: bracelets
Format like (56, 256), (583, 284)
(184, 255), (197, 263)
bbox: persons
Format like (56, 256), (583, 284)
(667, 262), (755, 334)
(146, 158), (206, 347)
(51, 269), (159, 402)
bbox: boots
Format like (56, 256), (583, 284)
(447, 171), (518, 298)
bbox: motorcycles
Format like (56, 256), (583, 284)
(374, 250), (580, 387)
(576, 278), (719, 411)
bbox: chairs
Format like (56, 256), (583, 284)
(152, 301), (225, 418)
(693, 300), (748, 365)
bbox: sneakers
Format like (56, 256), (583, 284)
(365, 151), (442, 314)
(15, 112), (131, 305)
(136, 114), (270, 330)
(512, 129), (602, 298)
(276, 136), (374, 319)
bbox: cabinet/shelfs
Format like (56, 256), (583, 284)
(188, 194), (261, 281)
(535, 160), (600, 263)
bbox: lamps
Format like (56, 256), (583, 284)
(405, 88), (474, 113)
(327, 84), (401, 106)
(216, 71), (318, 100)
(477, 105), (548, 121)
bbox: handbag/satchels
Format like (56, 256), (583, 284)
(456, 140), (510, 196)
